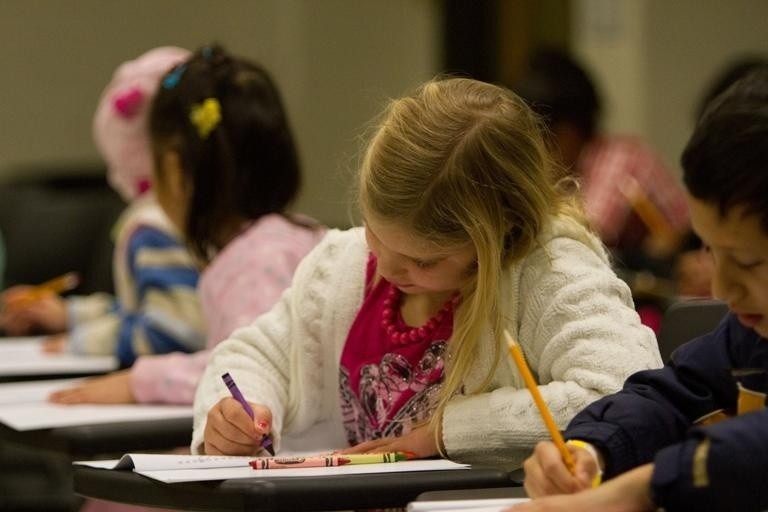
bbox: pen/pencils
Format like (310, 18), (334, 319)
(0, 271), (81, 314)
(623, 179), (703, 274)
(248, 450), (416, 469)
(503, 328), (592, 489)
(221, 372), (275, 456)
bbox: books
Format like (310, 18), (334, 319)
(1, 333), (122, 377)
(0, 380), (196, 434)
(69, 450), (471, 485)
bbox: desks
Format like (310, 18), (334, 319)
(1, 345), (523, 512)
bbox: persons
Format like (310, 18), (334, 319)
(637, 226), (716, 338)
(0, 283), (69, 337)
(490, 41), (689, 259)
(48, 50), (330, 510)
(30, 42), (212, 376)
(501, 69), (768, 510)
(188, 76), (669, 460)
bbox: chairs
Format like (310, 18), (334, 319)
(661, 298), (729, 360)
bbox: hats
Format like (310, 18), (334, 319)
(93, 43), (192, 192)
